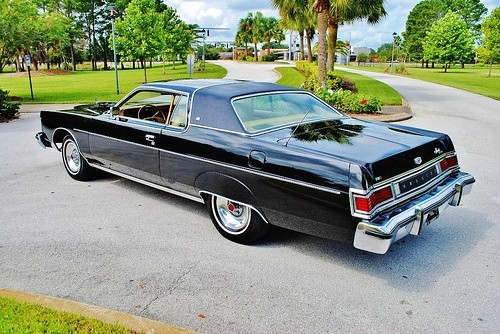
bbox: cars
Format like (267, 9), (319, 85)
(34, 78), (476, 255)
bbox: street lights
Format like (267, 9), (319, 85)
(391, 31), (397, 68)
(106, 6), (120, 95)
(201, 28), (206, 70)
